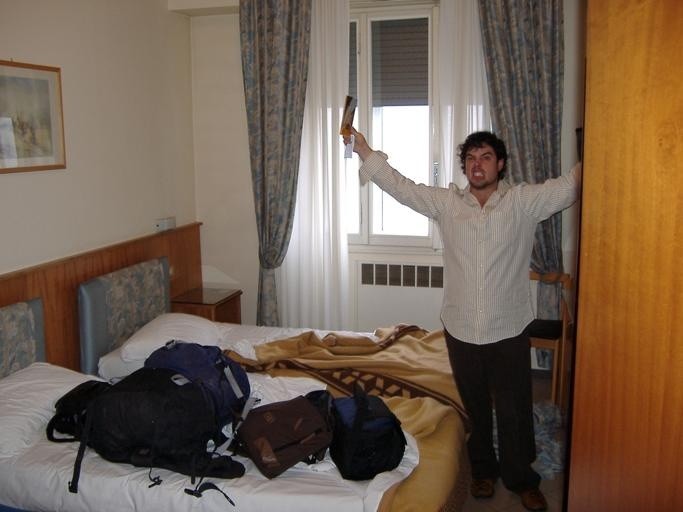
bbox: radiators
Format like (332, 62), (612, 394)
(354, 260), (538, 370)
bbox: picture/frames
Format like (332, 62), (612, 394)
(0, 60), (66, 174)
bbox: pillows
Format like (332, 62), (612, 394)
(122, 313), (215, 361)
(0, 362), (104, 463)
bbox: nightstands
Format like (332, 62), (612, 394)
(170, 287), (243, 324)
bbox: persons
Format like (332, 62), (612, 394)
(341, 126), (583, 510)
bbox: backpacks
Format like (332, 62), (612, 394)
(45, 379), (246, 477)
(143, 339), (250, 427)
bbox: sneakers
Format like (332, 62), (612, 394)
(470, 478), (496, 497)
(517, 487), (547, 512)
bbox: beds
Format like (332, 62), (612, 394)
(0, 297), (465, 512)
(78, 256), (475, 435)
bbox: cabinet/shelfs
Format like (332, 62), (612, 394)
(568, 1), (683, 511)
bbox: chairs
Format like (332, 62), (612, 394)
(522, 273), (574, 423)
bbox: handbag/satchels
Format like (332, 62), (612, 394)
(328, 382), (408, 482)
(235, 389), (335, 482)
